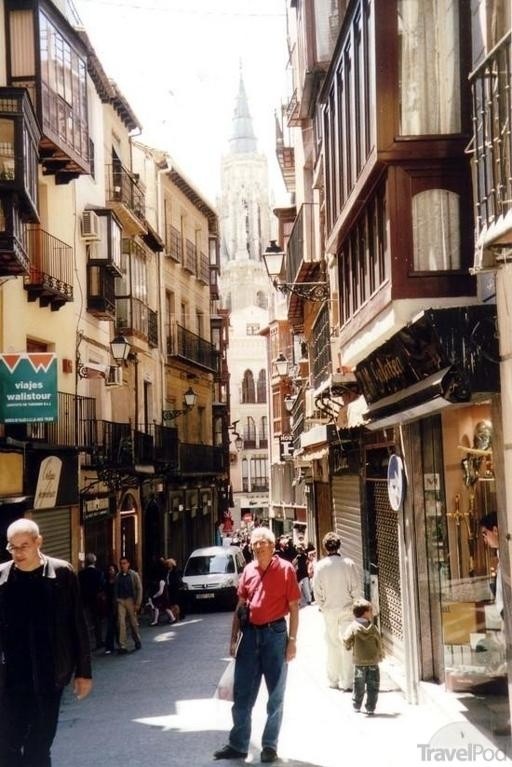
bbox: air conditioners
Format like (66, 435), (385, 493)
(104, 365), (123, 389)
(81, 211), (102, 244)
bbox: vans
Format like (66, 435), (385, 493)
(183, 545), (247, 607)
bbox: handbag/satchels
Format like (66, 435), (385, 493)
(237, 604), (253, 635)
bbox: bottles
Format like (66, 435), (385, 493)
(475, 630), (502, 663)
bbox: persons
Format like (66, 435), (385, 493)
(0, 518), (93, 766)
(81, 552), (184, 655)
(221, 520), (316, 607)
(479, 512), (511, 735)
(312, 531), (361, 692)
(214, 526), (303, 762)
(343, 600), (386, 715)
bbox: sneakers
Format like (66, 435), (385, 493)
(259, 747), (280, 764)
(212, 744), (250, 761)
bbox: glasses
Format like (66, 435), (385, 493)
(6, 540), (37, 553)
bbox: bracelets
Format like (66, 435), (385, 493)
(290, 637), (296, 641)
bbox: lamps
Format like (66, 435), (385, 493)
(108, 330), (132, 372)
(263, 239), (330, 303)
(271, 353), (303, 386)
(160, 387), (199, 420)
(282, 392), (297, 415)
